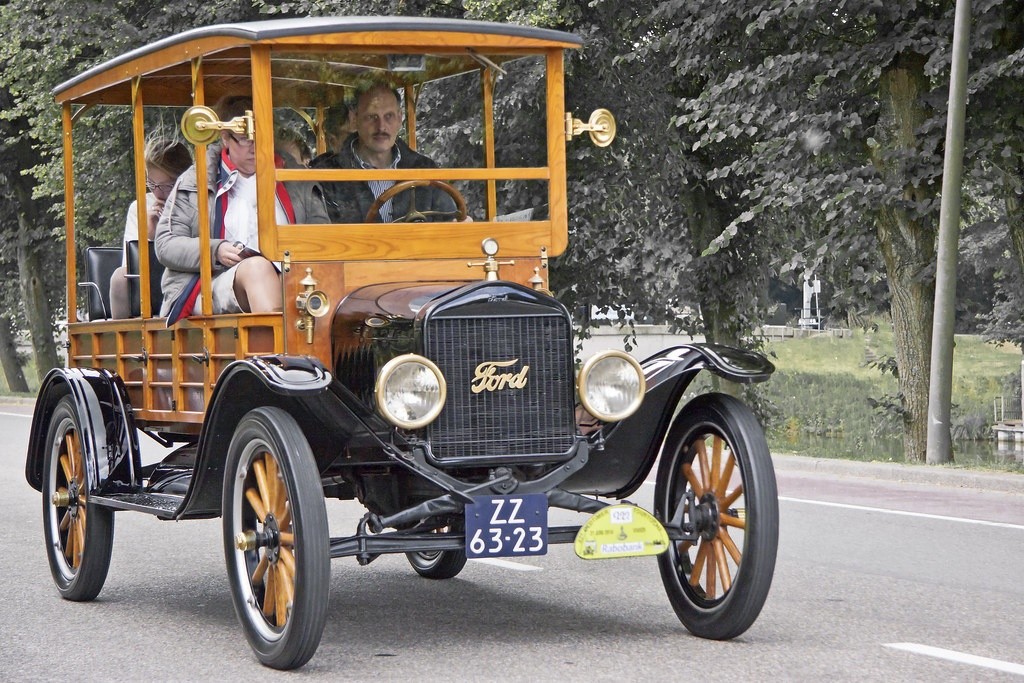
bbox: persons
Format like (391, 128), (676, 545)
(314, 79), (474, 225)
(110, 99), (355, 317)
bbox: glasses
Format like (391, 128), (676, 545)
(228, 133), (255, 147)
(145, 179), (175, 193)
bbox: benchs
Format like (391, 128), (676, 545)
(78, 238), (167, 322)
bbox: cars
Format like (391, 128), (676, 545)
(20, 18), (783, 673)
